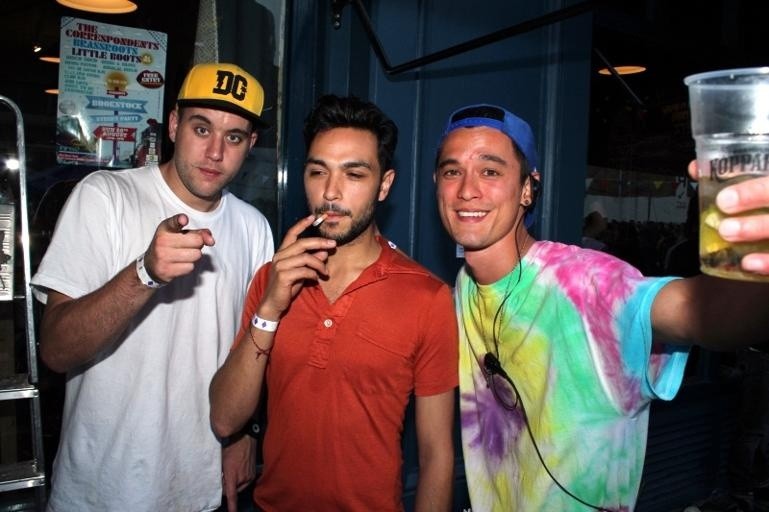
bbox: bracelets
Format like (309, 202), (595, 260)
(136, 254), (168, 290)
(248, 322), (275, 359)
(240, 419), (263, 439)
(253, 313), (279, 333)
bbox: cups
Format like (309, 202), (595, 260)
(685, 65), (769, 284)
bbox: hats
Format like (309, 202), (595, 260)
(171, 62), (268, 129)
(434, 104), (539, 232)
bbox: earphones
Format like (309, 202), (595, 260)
(528, 174), (540, 215)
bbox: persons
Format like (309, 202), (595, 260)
(582, 209), (612, 254)
(30, 64), (276, 512)
(209, 95), (460, 512)
(430, 102), (769, 510)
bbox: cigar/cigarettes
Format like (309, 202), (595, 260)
(312, 213), (328, 227)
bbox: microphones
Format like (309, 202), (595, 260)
(483, 352), (506, 378)
